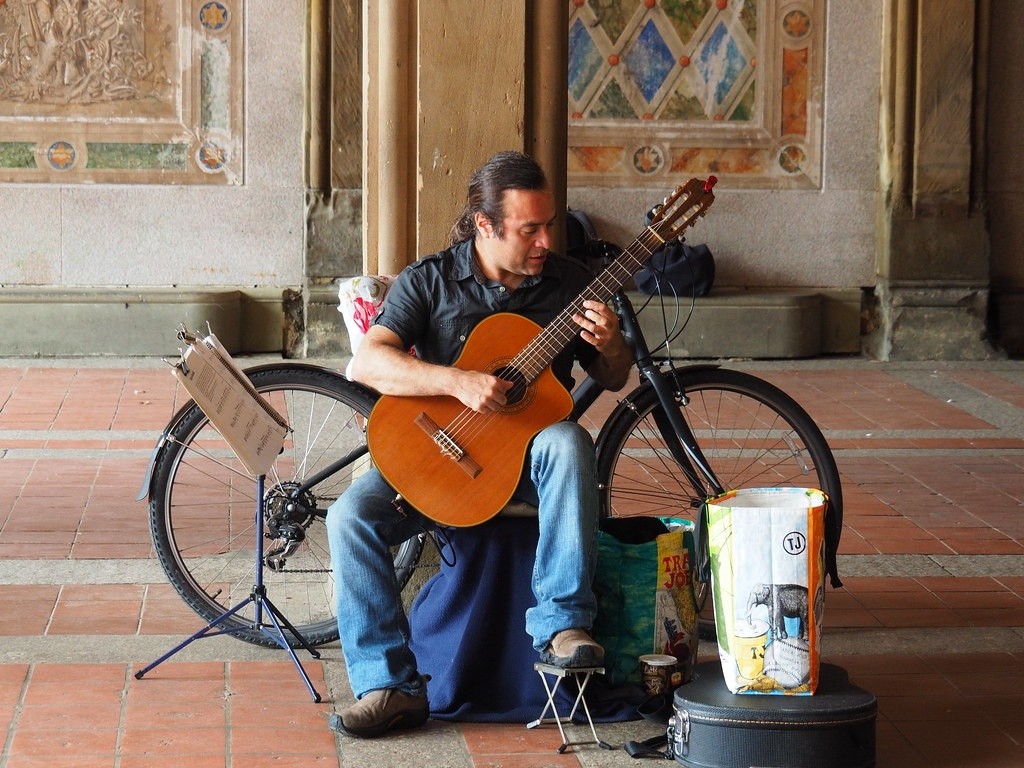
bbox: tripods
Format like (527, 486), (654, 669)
(134, 320), (323, 703)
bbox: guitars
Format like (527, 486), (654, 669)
(366, 174), (717, 532)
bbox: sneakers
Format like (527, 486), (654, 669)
(329, 687), (430, 739)
(539, 629), (605, 668)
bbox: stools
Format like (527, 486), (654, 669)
(526, 662), (612, 754)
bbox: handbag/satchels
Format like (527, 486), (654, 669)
(693, 486), (844, 698)
(595, 515), (698, 702)
(633, 239), (715, 296)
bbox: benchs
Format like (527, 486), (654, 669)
(606, 290), (824, 359)
(0, 288), (241, 356)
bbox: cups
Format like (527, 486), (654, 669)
(637, 655), (678, 695)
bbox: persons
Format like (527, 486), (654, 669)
(327, 151), (632, 741)
